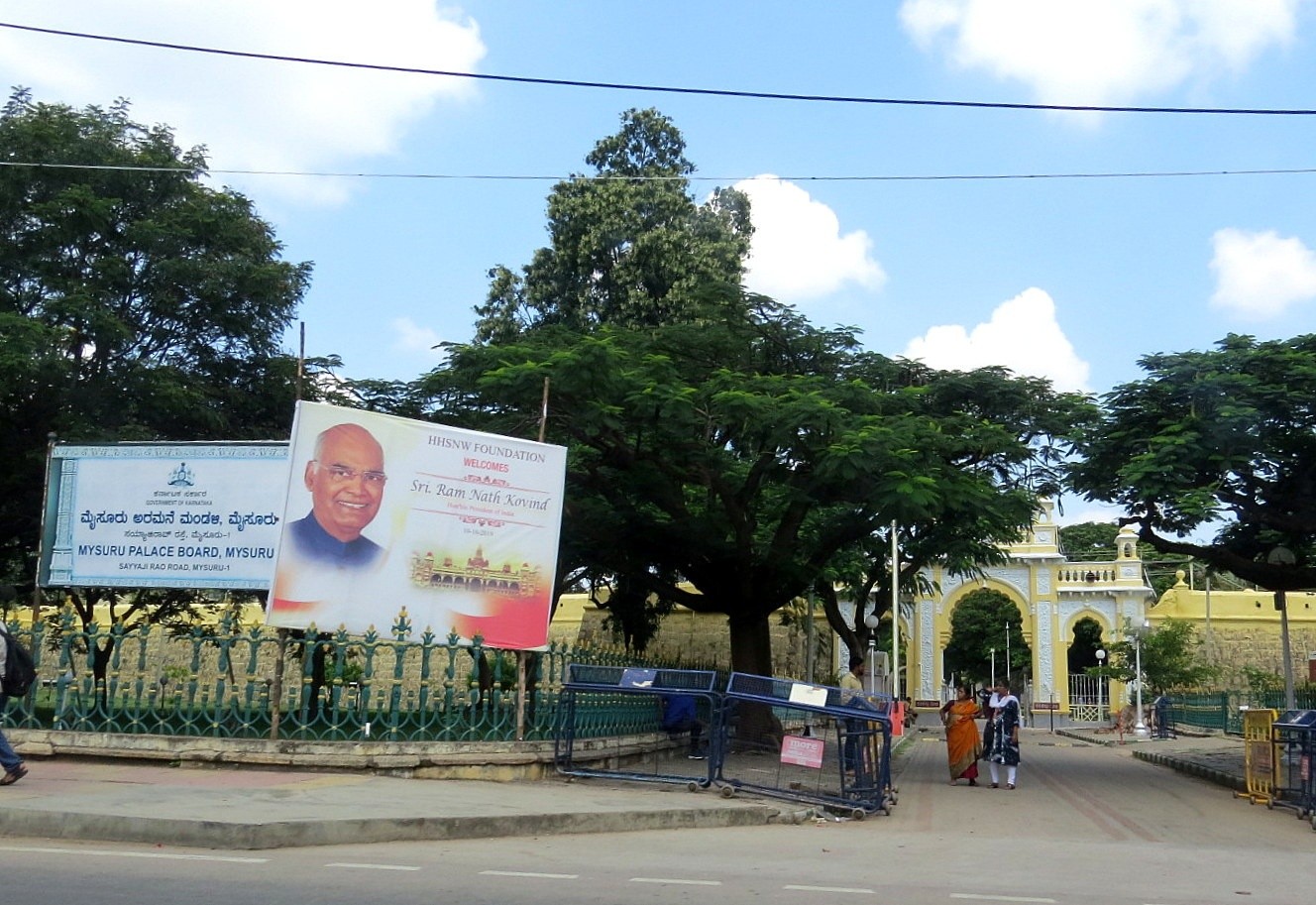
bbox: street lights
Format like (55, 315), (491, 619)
(864, 615), (878, 694)
(1268, 546), (1303, 765)
(1129, 620), (1150, 734)
(1096, 650), (1105, 721)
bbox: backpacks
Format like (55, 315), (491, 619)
(0, 626), (36, 697)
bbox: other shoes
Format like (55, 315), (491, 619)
(987, 783), (999, 788)
(845, 771), (855, 775)
(969, 782), (978, 786)
(0, 763), (28, 785)
(1005, 783), (1015, 789)
(688, 753), (703, 759)
(950, 780), (956, 785)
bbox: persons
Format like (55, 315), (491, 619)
(982, 679), (1020, 790)
(653, 683), (712, 759)
(1155, 696), (1172, 739)
(939, 685), (982, 787)
(904, 696), (918, 727)
(0, 618), (29, 787)
(288, 423), (387, 569)
(839, 656), (867, 771)
(729, 701), (785, 759)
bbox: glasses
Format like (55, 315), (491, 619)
(320, 464), (388, 486)
